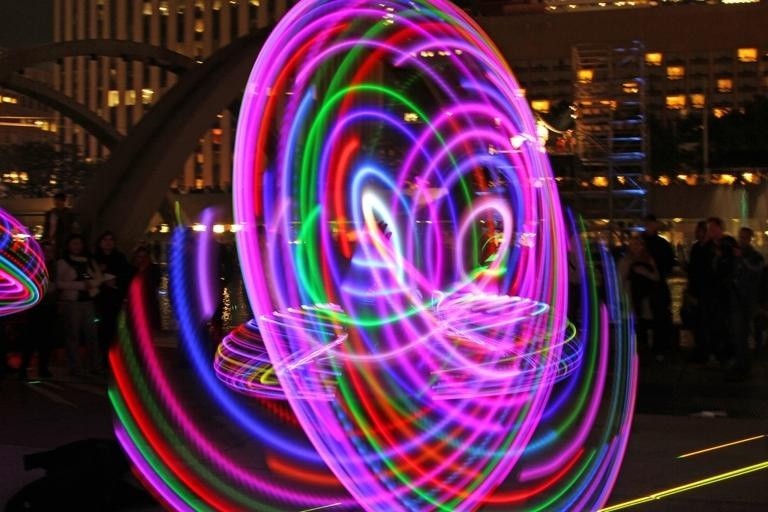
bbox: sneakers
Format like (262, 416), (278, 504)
(698, 360), (720, 370)
(724, 366), (750, 382)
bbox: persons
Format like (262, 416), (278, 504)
(2, 192), (254, 383)
(545, 213), (768, 384)
